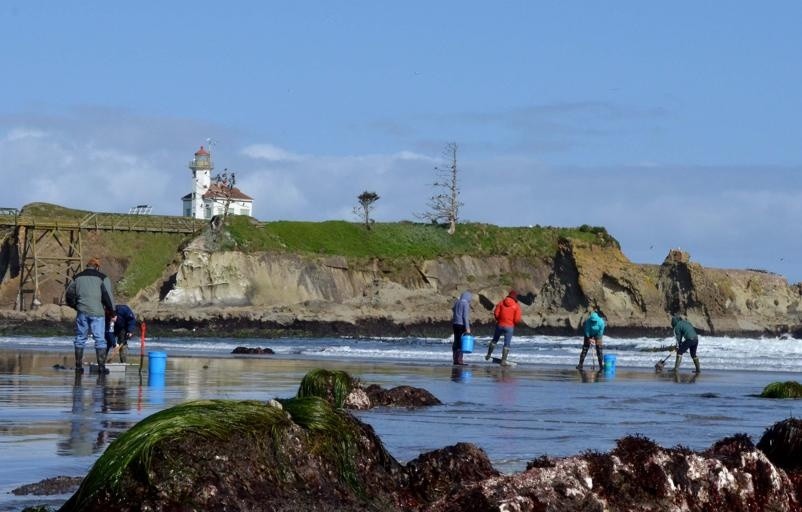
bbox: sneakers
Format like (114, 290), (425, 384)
(576, 365), (583, 369)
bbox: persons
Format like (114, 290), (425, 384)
(674, 373), (699, 386)
(55, 376), (114, 459)
(669, 314), (703, 372)
(574, 311), (607, 372)
(64, 257), (118, 375)
(105, 373), (130, 443)
(492, 369), (519, 422)
(483, 289), (523, 368)
(105, 302), (139, 365)
(577, 369), (603, 383)
(444, 368), (478, 423)
(451, 291), (473, 366)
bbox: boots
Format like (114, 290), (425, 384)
(501, 348), (512, 365)
(96, 348), (109, 373)
(119, 345), (128, 362)
(454, 350), (468, 365)
(486, 342), (496, 360)
(692, 357), (699, 372)
(673, 356), (681, 370)
(75, 347), (84, 372)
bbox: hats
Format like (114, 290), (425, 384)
(507, 291), (518, 302)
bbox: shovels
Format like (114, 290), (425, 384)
(655, 349), (676, 369)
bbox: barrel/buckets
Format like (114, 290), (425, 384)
(603, 354), (616, 367)
(147, 374), (166, 404)
(460, 332), (474, 353)
(460, 369), (473, 384)
(603, 368), (616, 380)
(148, 351), (168, 374)
